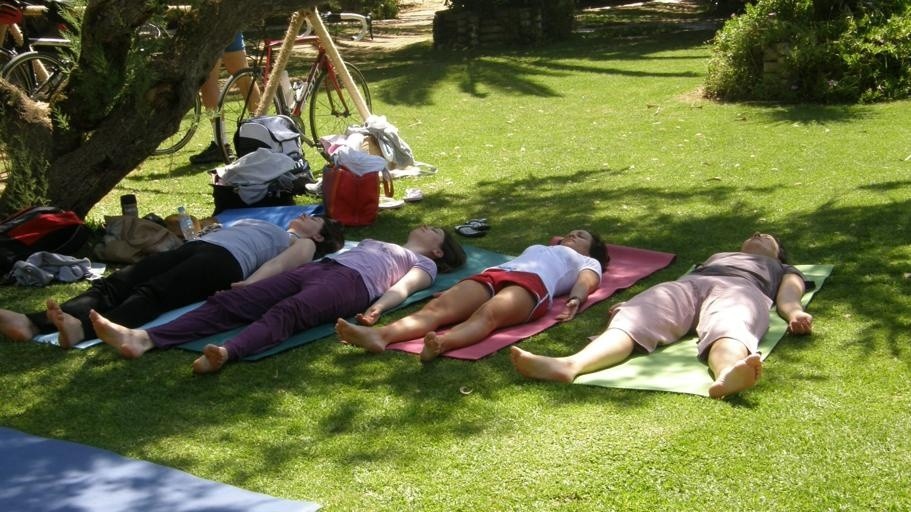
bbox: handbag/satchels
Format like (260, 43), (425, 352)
(92, 214), (183, 265)
(323, 161), (394, 227)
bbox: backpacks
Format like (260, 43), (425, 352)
(0, 204), (91, 285)
(233, 114), (303, 161)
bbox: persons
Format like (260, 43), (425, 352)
(169, 0), (261, 164)
(88, 225), (467, 375)
(0, 211), (347, 349)
(334, 227), (610, 364)
(510, 230), (816, 400)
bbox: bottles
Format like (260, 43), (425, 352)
(121, 195), (140, 218)
(177, 206), (195, 239)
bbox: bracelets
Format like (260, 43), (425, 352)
(565, 296), (583, 303)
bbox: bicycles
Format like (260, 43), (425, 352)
(1, 0), (202, 166)
(213, 13), (378, 165)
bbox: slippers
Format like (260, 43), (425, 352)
(402, 188), (423, 203)
(378, 193), (404, 209)
(464, 218), (491, 229)
(454, 224), (486, 237)
